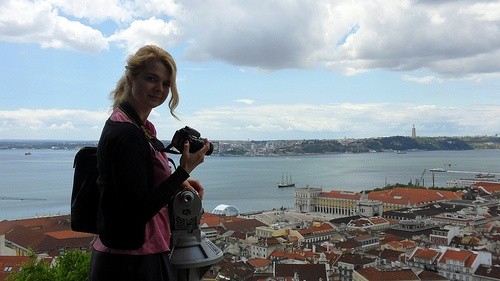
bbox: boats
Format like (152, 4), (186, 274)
(278, 174), (295, 187)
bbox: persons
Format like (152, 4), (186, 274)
(89, 45), (210, 281)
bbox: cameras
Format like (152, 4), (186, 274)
(171, 126), (213, 156)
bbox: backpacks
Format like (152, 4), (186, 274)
(71, 147), (100, 234)
